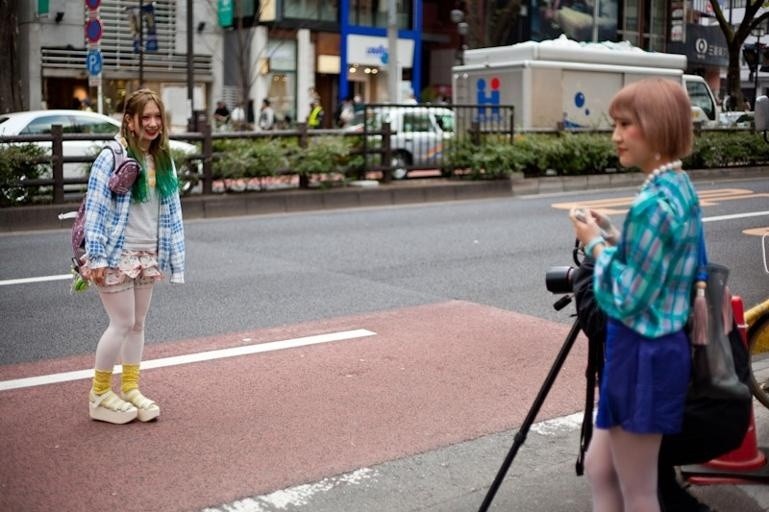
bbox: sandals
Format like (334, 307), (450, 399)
(120, 390), (161, 424)
(88, 388), (138, 426)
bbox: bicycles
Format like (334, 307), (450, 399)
(732, 286), (768, 406)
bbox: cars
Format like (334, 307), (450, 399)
(338, 105), (453, 180)
(0, 108), (203, 207)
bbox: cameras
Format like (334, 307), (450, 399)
(544, 263), (578, 295)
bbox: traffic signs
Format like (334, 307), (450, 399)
(82, 1), (104, 121)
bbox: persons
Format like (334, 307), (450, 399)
(230, 102), (245, 124)
(568, 79), (755, 511)
(213, 101), (232, 128)
(336, 95), (355, 128)
(69, 89), (185, 425)
(256, 97), (274, 130)
(307, 100), (325, 128)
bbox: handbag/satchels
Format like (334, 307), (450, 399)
(694, 258), (744, 395)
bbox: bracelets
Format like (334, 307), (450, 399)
(583, 235), (605, 257)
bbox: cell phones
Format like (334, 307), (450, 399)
(574, 210), (611, 240)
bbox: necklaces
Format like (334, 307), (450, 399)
(639, 158), (684, 187)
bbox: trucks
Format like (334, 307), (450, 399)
(451, 34), (722, 136)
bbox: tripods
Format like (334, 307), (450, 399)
(476, 307), (608, 512)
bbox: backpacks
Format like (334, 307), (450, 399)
(69, 138), (140, 291)
